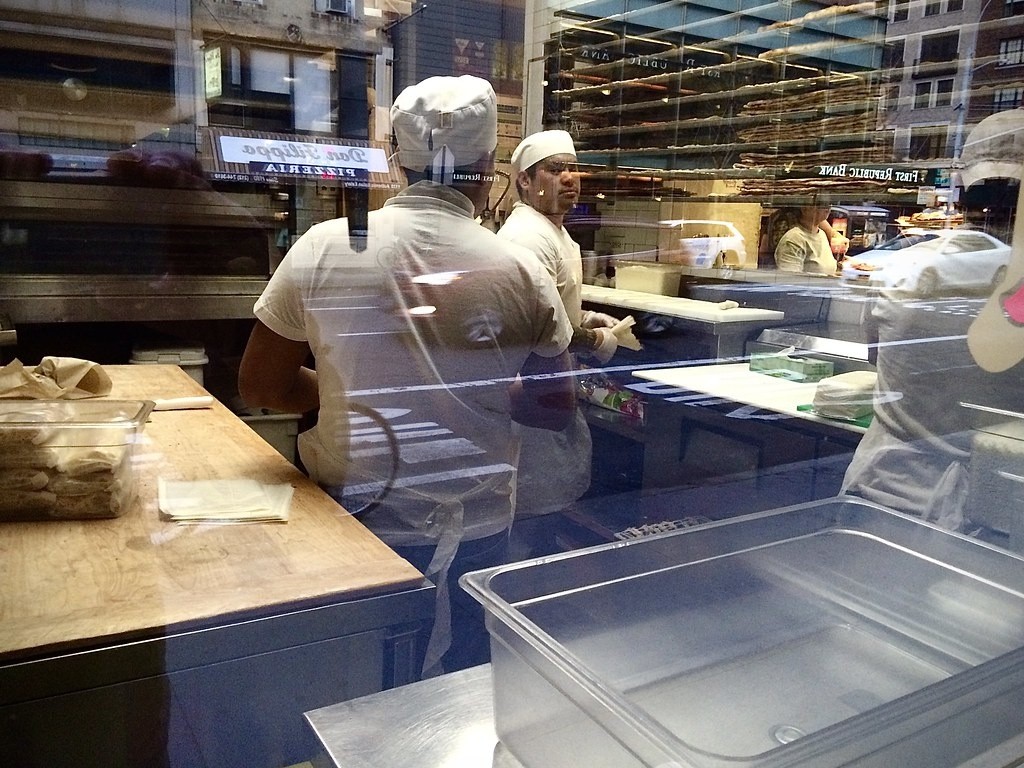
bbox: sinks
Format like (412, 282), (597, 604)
(459, 495), (1024, 768)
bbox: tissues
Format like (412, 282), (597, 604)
(749, 346), (834, 384)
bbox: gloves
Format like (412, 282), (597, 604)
(580, 310), (620, 332)
(590, 328), (618, 366)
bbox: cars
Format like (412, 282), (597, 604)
(841, 225), (1011, 300)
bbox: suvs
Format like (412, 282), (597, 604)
(565, 217), (695, 337)
(654, 219), (750, 275)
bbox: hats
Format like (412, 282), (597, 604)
(957, 109), (1024, 193)
(391, 75), (498, 173)
(510, 129), (576, 177)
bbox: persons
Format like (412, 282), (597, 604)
(774, 186), (837, 277)
(839, 109), (1024, 518)
(238, 74), (621, 676)
